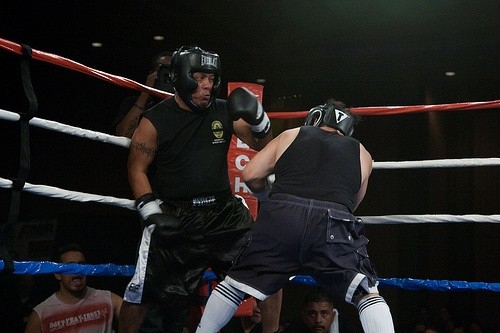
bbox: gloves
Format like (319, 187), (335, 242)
(136, 194), (184, 250)
(227, 84), (270, 138)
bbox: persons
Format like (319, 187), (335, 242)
(196, 98), (395, 333)
(118, 44), (283, 333)
(115, 52), (171, 140)
(217, 293), (263, 333)
(25, 244), (123, 333)
(279, 291), (364, 333)
(414, 304), (483, 333)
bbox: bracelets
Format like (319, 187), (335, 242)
(133, 104), (144, 110)
